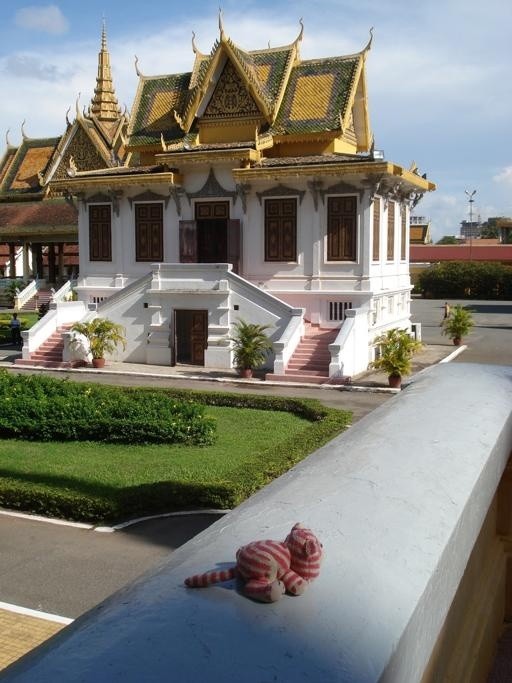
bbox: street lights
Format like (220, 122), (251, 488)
(463, 187), (478, 262)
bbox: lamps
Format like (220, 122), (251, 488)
(67, 166), (78, 177)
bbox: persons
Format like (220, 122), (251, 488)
(439, 301), (450, 327)
(9, 312), (23, 345)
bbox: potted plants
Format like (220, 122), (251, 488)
(225, 320), (274, 377)
(438, 302), (473, 345)
(368, 327), (422, 388)
(67, 317), (128, 367)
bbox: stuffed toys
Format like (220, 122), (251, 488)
(183, 520), (326, 603)
